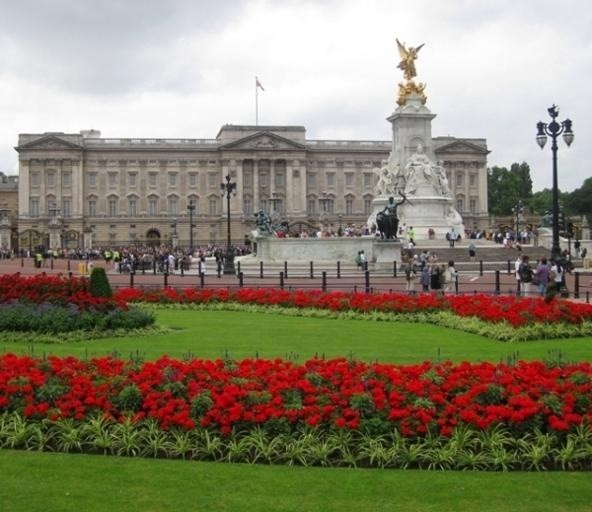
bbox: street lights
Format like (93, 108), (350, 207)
(220, 174), (238, 274)
(512, 198), (524, 243)
(537, 104), (574, 267)
(186, 200), (197, 254)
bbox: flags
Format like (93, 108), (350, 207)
(257, 80), (264, 90)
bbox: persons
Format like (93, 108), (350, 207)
(277, 222), (376, 238)
(380, 196), (406, 229)
(253, 211), (273, 234)
(399, 224), (592, 297)
(405, 48), (417, 79)
(355, 251), (368, 271)
(1, 249), (30, 261)
(33, 235), (253, 278)
